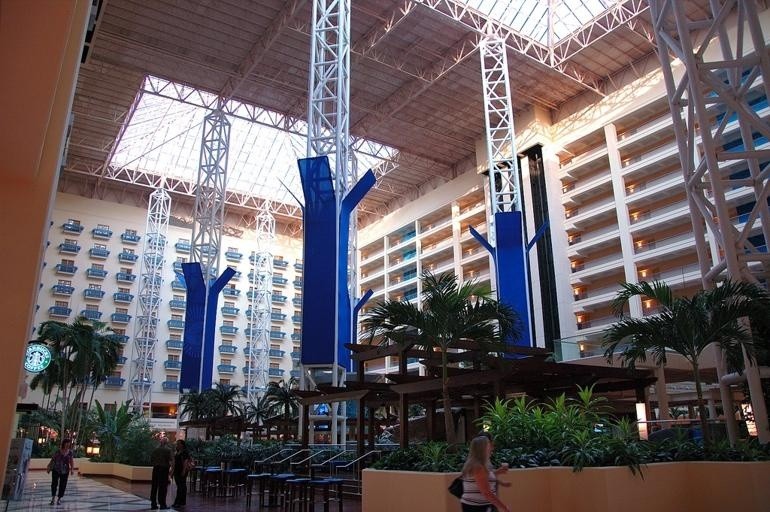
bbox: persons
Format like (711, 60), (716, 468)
(48, 439), (74, 505)
(461, 436), (512, 512)
(475, 431), (512, 512)
(150, 438), (175, 510)
(171, 440), (192, 508)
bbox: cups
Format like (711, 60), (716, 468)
(500, 461), (508, 473)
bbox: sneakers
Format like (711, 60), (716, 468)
(51, 497), (61, 505)
(151, 504), (188, 510)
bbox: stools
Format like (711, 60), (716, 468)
(191, 464), (346, 512)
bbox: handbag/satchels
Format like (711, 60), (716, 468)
(183, 459), (196, 471)
(49, 460), (54, 471)
(448, 475), (464, 499)
